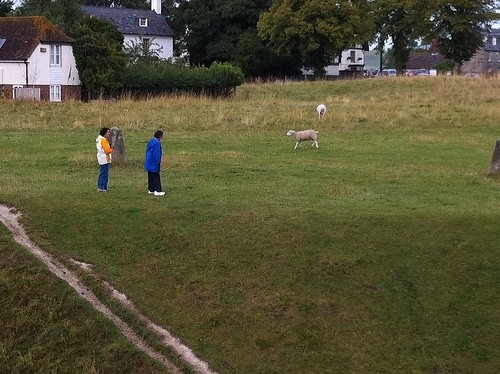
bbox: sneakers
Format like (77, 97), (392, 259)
(147, 191), (154, 194)
(154, 191), (166, 196)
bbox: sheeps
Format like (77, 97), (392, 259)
(316, 104), (326, 120)
(286, 130), (320, 149)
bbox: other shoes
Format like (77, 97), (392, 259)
(97, 188), (107, 192)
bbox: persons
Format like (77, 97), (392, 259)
(96, 127), (114, 192)
(145, 130), (166, 196)
